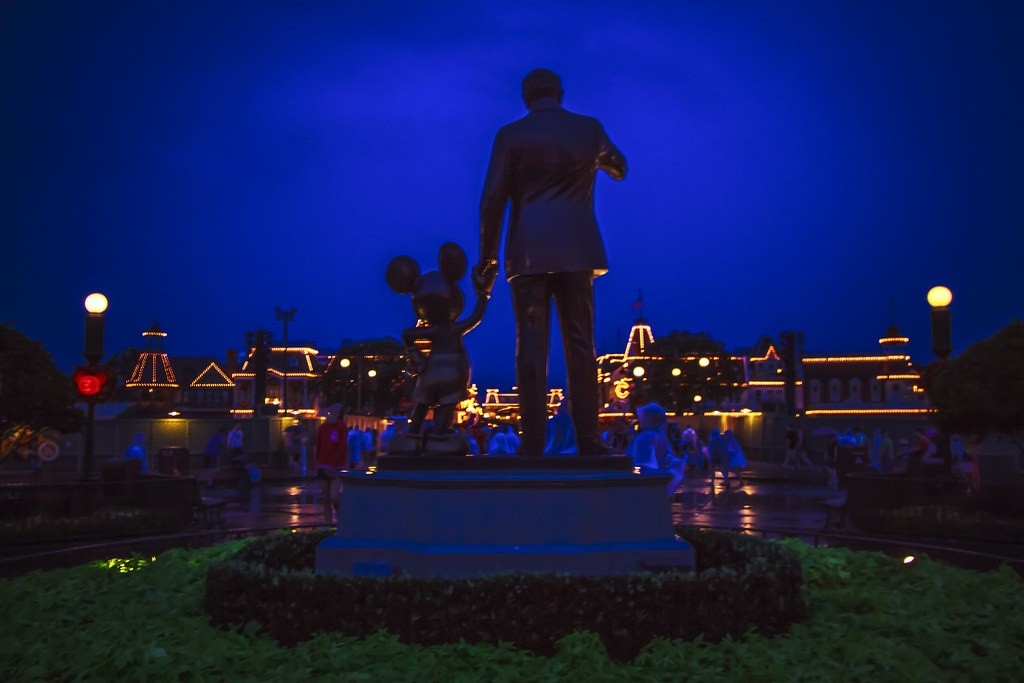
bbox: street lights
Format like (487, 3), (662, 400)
(74, 290), (112, 479)
(272, 307), (301, 416)
(927, 286), (952, 469)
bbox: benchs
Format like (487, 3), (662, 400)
(820, 472), (967, 538)
(188, 474), (230, 532)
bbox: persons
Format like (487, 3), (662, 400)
(315, 399), (351, 529)
(476, 67), (629, 456)
(31, 398), (1023, 489)
